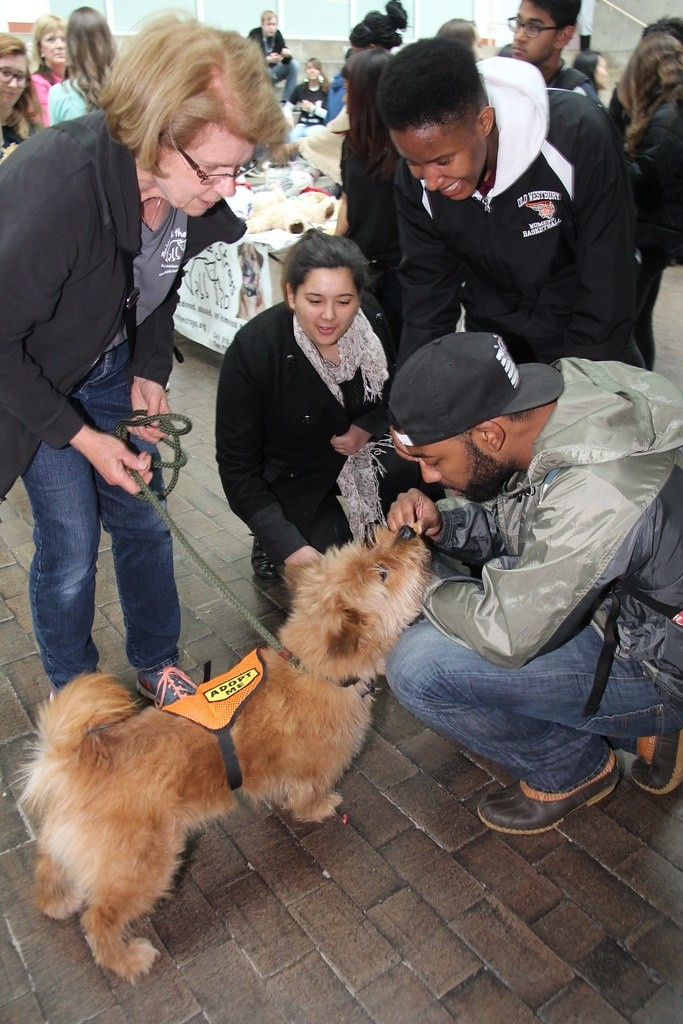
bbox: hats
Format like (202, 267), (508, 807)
(388, 332), (564, 446)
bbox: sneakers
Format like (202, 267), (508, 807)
(137, 663), (199, 710)
(251, 536), (282, 583)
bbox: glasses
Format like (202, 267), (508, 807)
(508, 17), (560, 38)
(176, 143), (259, 185)
(0, 68), (31, 89)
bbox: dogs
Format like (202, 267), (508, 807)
(6, 525), (432, 986)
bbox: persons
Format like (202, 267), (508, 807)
(573, 49), (610, 96)
(608, 17), (683, 372)
(498, 0), (605, 111)
(0, 31), (45, 159)
(376, 38), (635, 501)
(282, 57), (329, 142)
(213, 229), (444, 589)
(435, 17), (485, 62)
(47, 5), (118, 128)
(0, 8), (297, 712)
(386, 328), (683, 832)
(29, 15), (73, 130)
(324, 0), (408, 237)
(243, 10), (298, 108)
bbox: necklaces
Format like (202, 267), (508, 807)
(137, 198), (162, 229)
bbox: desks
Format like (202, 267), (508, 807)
(175, 243), (291, 356)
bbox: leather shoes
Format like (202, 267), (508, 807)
(631, 729), (683, 794)
(477, 748), (619, 834)
